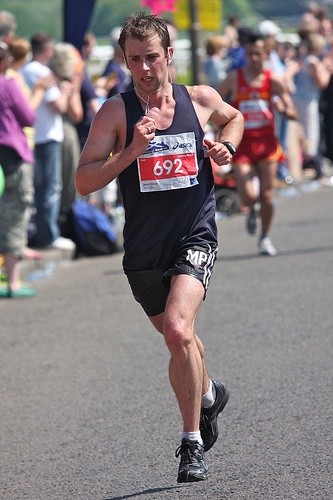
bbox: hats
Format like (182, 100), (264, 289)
(258, 20), (280, 35)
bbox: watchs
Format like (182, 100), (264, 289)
(223, 141), (236, 155)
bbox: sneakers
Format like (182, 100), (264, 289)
(200, 380), (230, 452)
(258, 237), (276, 256)
(313, 155), (330, 180)
(247, 213), (257, 235)
(177, 438), (209, 482)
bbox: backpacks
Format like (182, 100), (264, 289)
(69, 203), (123, 260)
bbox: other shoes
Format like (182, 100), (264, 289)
(23, 247), (42, 259)
(9, 287), (38, 299)
(0, 288), (10, 297)
(48, 237), (75, 250)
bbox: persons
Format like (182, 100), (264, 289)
(74, 11), (245, 483)
(0, 1), (333, 298)
